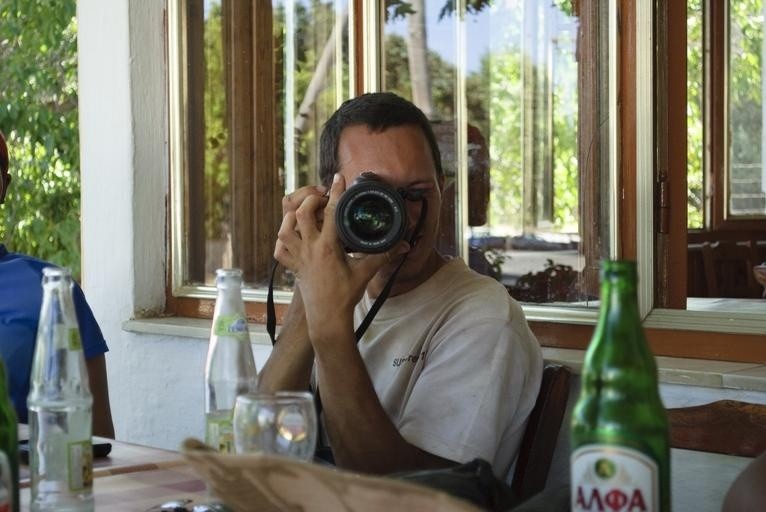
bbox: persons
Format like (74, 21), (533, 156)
(0, 123), (120, 447)
(258, 93), (545, 502)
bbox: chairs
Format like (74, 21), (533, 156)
(511, 362), (569, 511)
(666, 400), (765, 511)
(703, 240), (760, 298)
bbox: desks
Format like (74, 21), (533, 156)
(16, 424), (486, 512)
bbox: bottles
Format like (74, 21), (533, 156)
(204, 267), (259, 453)
(27, 265), (95, 512)
(0, 353), (21, 511)
(568, 259), (671, 510)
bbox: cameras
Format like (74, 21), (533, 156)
(334, 168), (409, 257)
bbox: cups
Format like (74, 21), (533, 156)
(232, 391), (318, 467)
